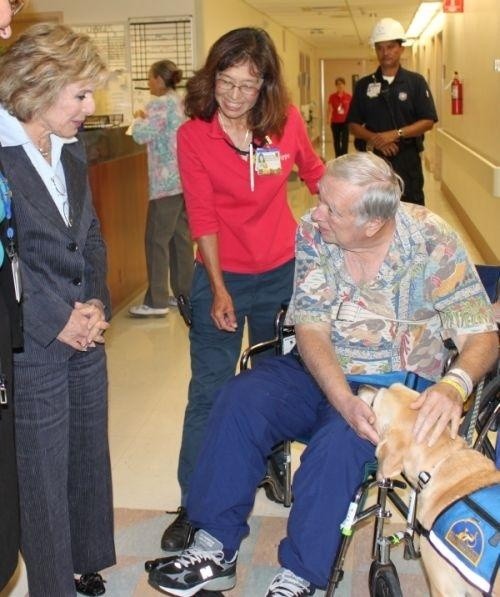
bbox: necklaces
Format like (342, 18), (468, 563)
(19, 119), (54, 162)
(218, 111), (250, 150)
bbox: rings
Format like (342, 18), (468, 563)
(375, 139), (377, 143)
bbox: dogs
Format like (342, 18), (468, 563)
(356, 382), (500, 597)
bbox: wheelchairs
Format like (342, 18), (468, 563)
(143, 263), (497, 596)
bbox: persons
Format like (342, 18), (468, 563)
(160, 25), (329, 553)
(0, 19), (116, 596)
(0, 0), (33, 597)
(326, 77), (352, 158)
(345, 16), (439, 206)
(147, 149), (499, 597)
(127, 59), (195, 316)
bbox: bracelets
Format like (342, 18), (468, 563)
(86, 299), (106, 314)
(439, 368), (474, 403)
(396, 128), (405, 140)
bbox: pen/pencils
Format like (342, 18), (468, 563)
(249, 144), (255, 192)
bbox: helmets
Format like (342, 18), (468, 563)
(371, 18), (406, 43)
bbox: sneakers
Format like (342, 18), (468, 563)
(265, 569), (315, 597)
(74, 507), (238, 597)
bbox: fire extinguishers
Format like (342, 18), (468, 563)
(444, 72), (463, 115)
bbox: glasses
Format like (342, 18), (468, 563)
(215, 79), (261, 94)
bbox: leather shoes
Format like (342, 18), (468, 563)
(128, 306), (168, 317)
